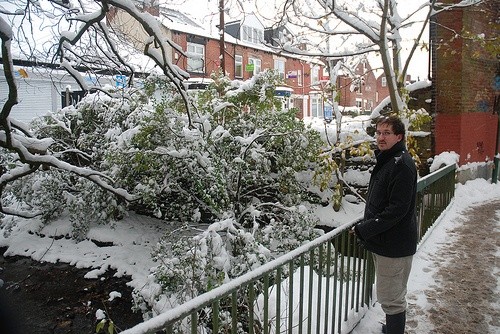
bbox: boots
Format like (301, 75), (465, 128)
(382, 311), (406, 334)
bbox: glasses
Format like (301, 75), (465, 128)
(374, 131), (395, 137)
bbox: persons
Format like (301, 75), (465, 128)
(353, 114), (419, 333)
